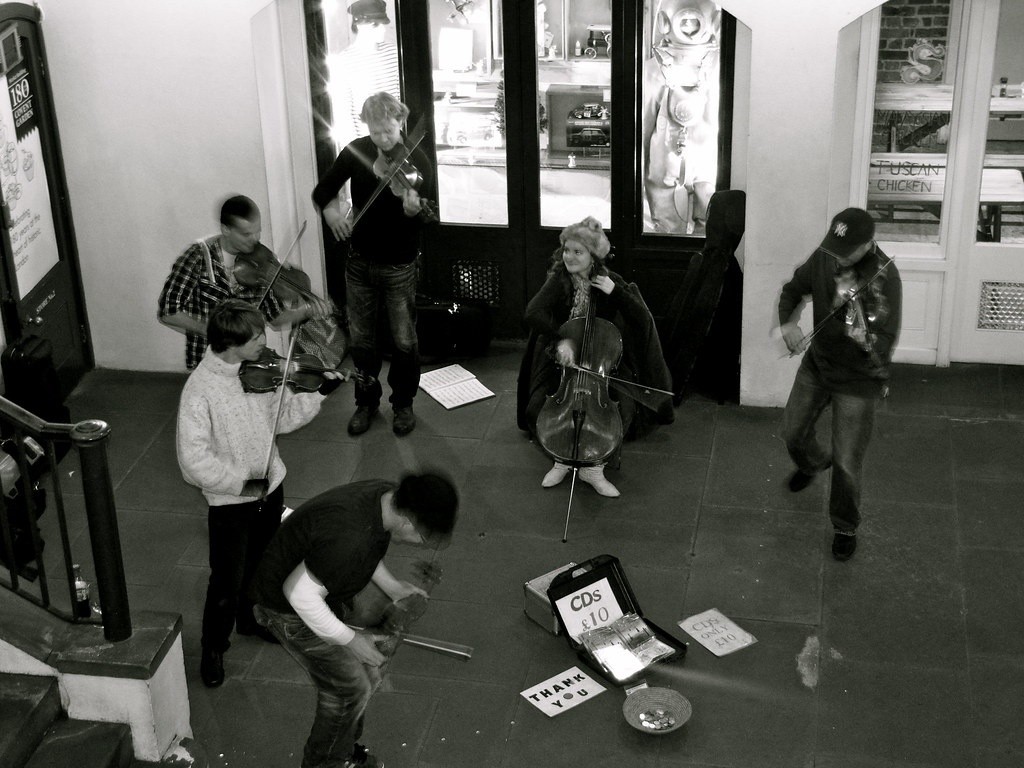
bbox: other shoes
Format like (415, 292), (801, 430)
(833, 530), (856, 562)
(785, 455), (834, 492)
(542, 458), (573, 488)
(578, 464), (621, 498)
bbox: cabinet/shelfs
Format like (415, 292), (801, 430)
(850, 0), (1024, 368)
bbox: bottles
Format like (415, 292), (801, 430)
(574, 40), (581, 56)
(72, 563), (95, 617)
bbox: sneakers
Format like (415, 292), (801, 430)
(302, 745), (385, 768)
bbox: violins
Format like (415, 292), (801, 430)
(372, 142), (443, 229)
(238, 345), (376, 393)
(831, 253), (880, 354)
(366, 558), (444, 653)
(232, 241), (359, 334)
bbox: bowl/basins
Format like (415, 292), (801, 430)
(622, 686), (692, 734)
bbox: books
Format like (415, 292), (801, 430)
(419, 364), (496, 409)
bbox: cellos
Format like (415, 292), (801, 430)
(535, 243), (625, 544)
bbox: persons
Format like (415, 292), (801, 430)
(251, 470), (459, 768)
(516, 216), (674, 497)
(159, 196), (334, 370)
(779, 208), (902, 559)
(311, 93), (435, 438)
(176, 300), (352, 689)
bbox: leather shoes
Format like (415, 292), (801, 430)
(393, 407), (416, 437)
(201, 644), (225, 686)
(236, 618), (281, 646)
(348, 403), (376, 435)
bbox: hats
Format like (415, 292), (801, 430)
(820, 207), (876, 260)
(400, 474), (459, 551)
(560, 215), (612, 261)
